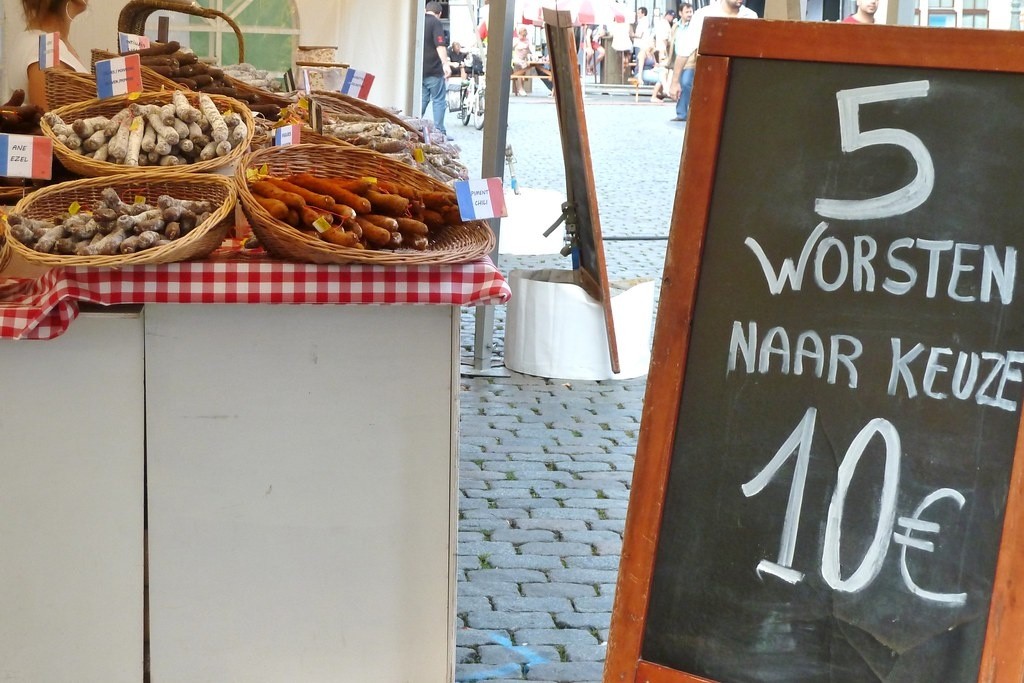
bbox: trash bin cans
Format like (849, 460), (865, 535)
(600, 35), (630, 85)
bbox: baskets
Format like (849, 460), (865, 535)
(44, 66), (169, 111)
(0, 172), (237, 268)
(117, 0), (426, 147)
(39, 90), (256, 172)
(91, 47), (191, 90)
(236, 143), (496, 265)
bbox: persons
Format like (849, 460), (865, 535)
(669, 0), (759, 100)
(421, 1), (456, 141)
(512, 28), (532, 97)
(842, 0), (880, 24)
(666, 2), (695, 121)
(610, 13), (633, 77)
(629, 6), (676, 102)
(585, 24), (607, 76)
(446, 41), (468, 79)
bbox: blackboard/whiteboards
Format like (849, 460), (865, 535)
(605, 15), (1024, 683)
(541, 5), (609, 300)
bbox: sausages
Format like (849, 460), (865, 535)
(0, 40), (465, 255)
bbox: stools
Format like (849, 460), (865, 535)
(616, 50), (630, 84)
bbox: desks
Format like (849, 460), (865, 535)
(511, 62), (550, 96)
(0, 257), (513, 683)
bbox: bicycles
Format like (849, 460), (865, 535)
(457, 61), (486, 130)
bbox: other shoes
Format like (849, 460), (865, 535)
(669, 116), (687, 123)
(446, 134), (454, 141)
(518, 89), (527, 97)
